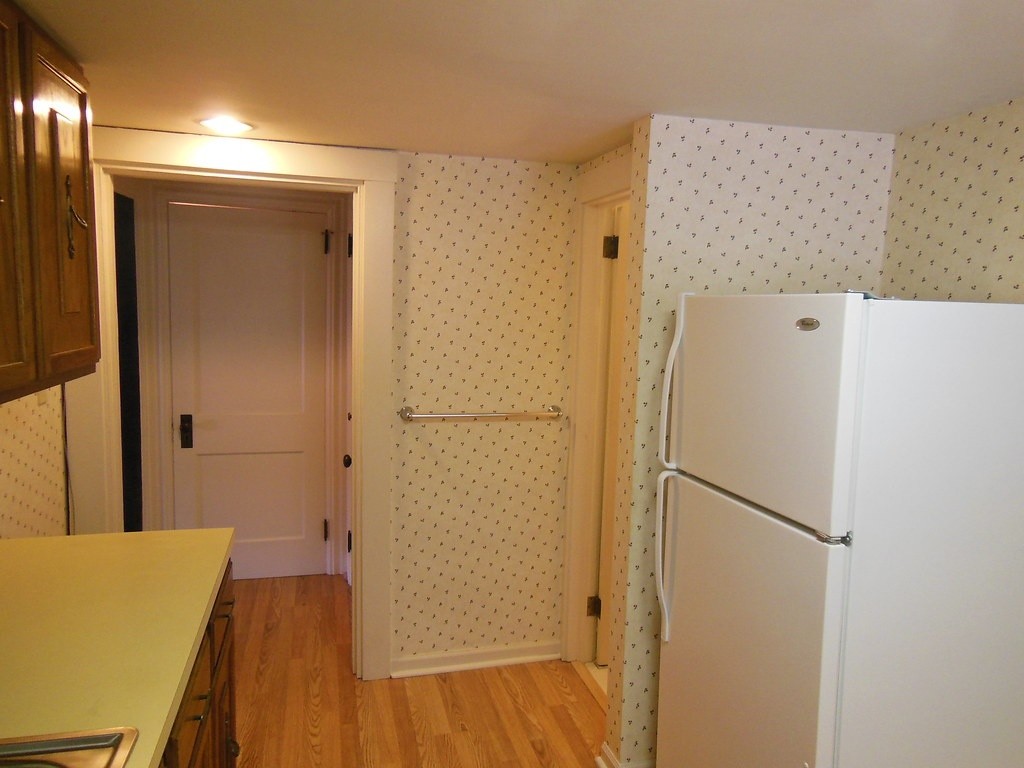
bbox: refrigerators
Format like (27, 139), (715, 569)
(653, 290), (1023, 768)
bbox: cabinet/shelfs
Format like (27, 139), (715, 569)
(1, 2), (28, 402)
(159, 560), (236, 768)
(28, 18), (100, 391)
